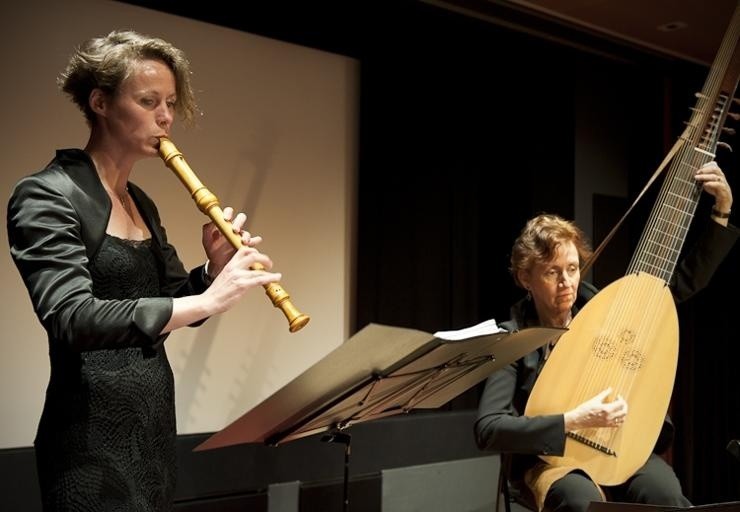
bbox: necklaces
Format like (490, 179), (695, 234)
(108, 184), (133, 211)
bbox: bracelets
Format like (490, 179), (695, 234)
(202, 259), (217, 283)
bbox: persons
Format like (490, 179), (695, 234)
(6, 30), (282, 511)
(473, 164), (733, 512)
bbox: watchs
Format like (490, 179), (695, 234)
(711, 205), (731, 218)
